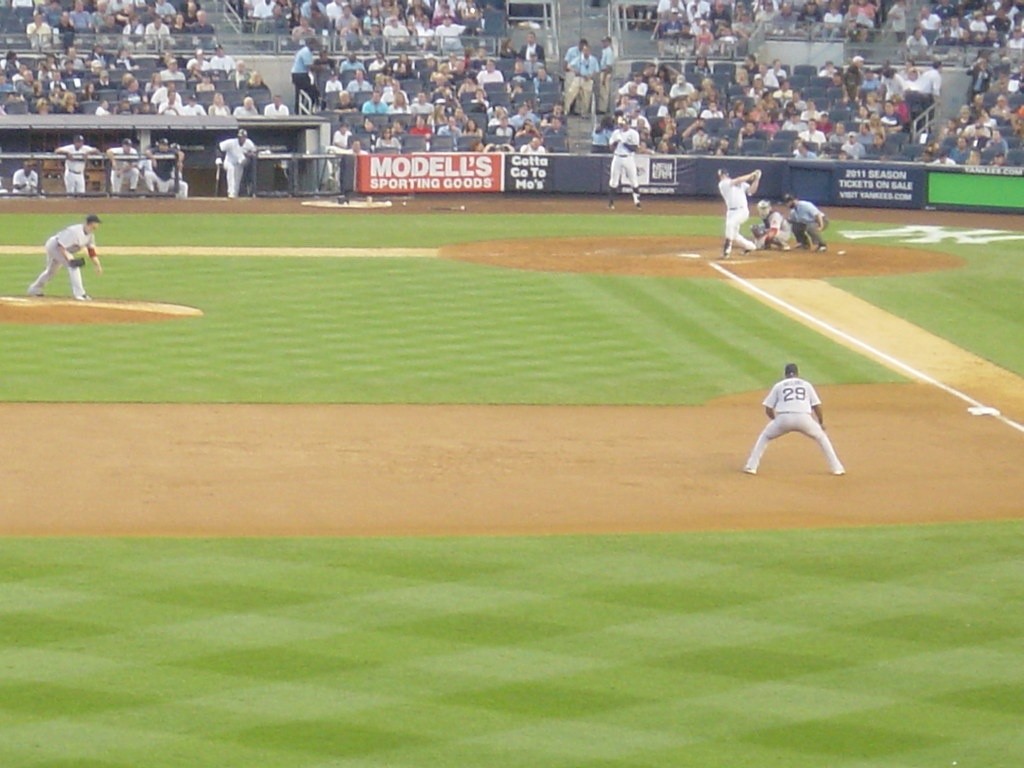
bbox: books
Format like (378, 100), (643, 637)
(0, 0), (1024, 199)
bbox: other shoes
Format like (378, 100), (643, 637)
(77, 293), (92, 301)
(818, 244), (827, 251)
(608, 200), (615, 210)
(742, 250), (750, 255)
(724, 253), (730, 259)
(636, 203), (641, 210)
(34, 292), (43, 297)
(743, 468), (757, 475)
(782, 245), (790, 250)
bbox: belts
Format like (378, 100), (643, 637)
(68, 169), (82, 174)
(730, 206), (743, 210)
(616, 153), (628, 157)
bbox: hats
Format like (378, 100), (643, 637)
(88, 216), (100, 223)
(717, 168), (727, 175)
(786, 364), (798, 376)
(785, 193), (793, 201)
(123, 138), (132, 145)
(238, 129), (247, 138)
(757, 199), (770, 208)
(74, 135), (84, 141)
(159, 138), (169, 144)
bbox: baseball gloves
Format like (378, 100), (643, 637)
(68, 258), (86, 268)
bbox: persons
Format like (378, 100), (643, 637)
(751, 199), (791, 251)
(781, 196), (829, 252)
(743, 365), (845, 475)
(27, 214), (102, 300)
(717, 167), (760, 262)
(607, 115), (643, 210)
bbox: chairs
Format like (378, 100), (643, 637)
(1, 0), (1024, 167)
